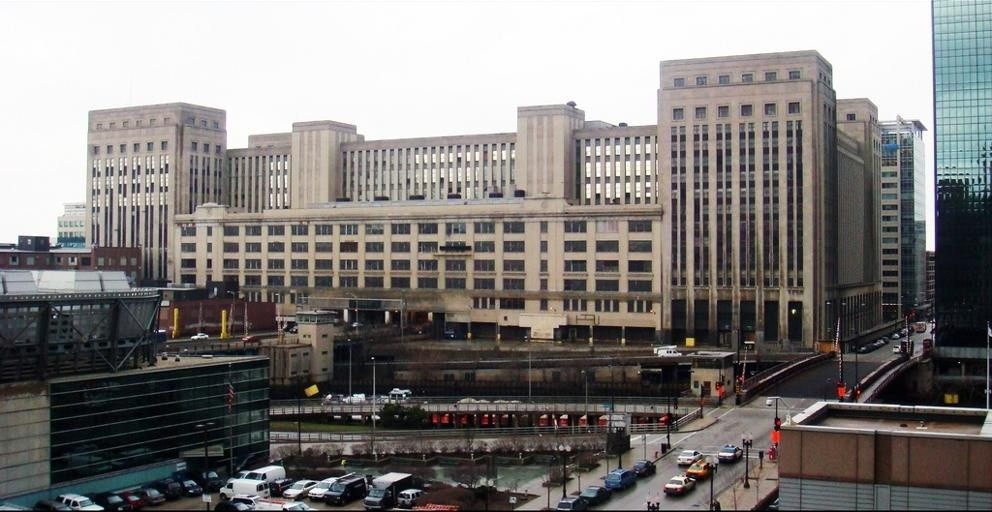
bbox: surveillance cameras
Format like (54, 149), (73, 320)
(766, 400), (773, 406)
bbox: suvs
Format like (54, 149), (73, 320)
(604, 468), (637, 490)
(556, 495), (589, 512)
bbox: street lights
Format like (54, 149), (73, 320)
(559, 442), (572, 498)
(344, 292), (359, 335)
(395, 295), (405, 344)
(579, 370), (590, 426)
(237, 290), (248, 335)
(614, 421), (628, 468)
(275, 293), (281, 334)
(195, 422), (215, 512)
(608, 363), (615, 413)
(368, 356), (378, 461)
(334, 318), (363, 330)
(281, 320), (298, 333)
(742, 430), (753, 490)
(347, 338), (353, 403)
(646, 491), (662, 511)
(227, 291), (236, 312)
(522, 335), (532, 403)
(854, 303), (869, 386)
(825, 301), (847, 382)
(704, 455), (720, 511)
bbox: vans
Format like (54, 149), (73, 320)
(657, 348), (682, 358)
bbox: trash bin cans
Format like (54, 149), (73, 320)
(662, 443), (666, 454)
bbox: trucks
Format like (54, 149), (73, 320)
(152, 329), (166, 342)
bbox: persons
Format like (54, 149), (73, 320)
(713, 498), (720, 511)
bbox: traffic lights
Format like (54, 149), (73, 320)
(855, 388), (863, 396)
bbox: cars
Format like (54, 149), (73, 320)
(633, 460), (657, 478)
(677, 450), (703, 466)
(719, 444), (743, 465)
(320, 393), (524, 405)
(687, 459), (714, 481)
(856, 318), (935, 354)
(191, 332), (209, 341)
(412, 319), (433, 336)
(664, 475), (696, 495)
(580, 485), (611, 506)
(444, 326), (464, 339)
(61, 437), (156, 474)
(241, 335), (257, 343)
